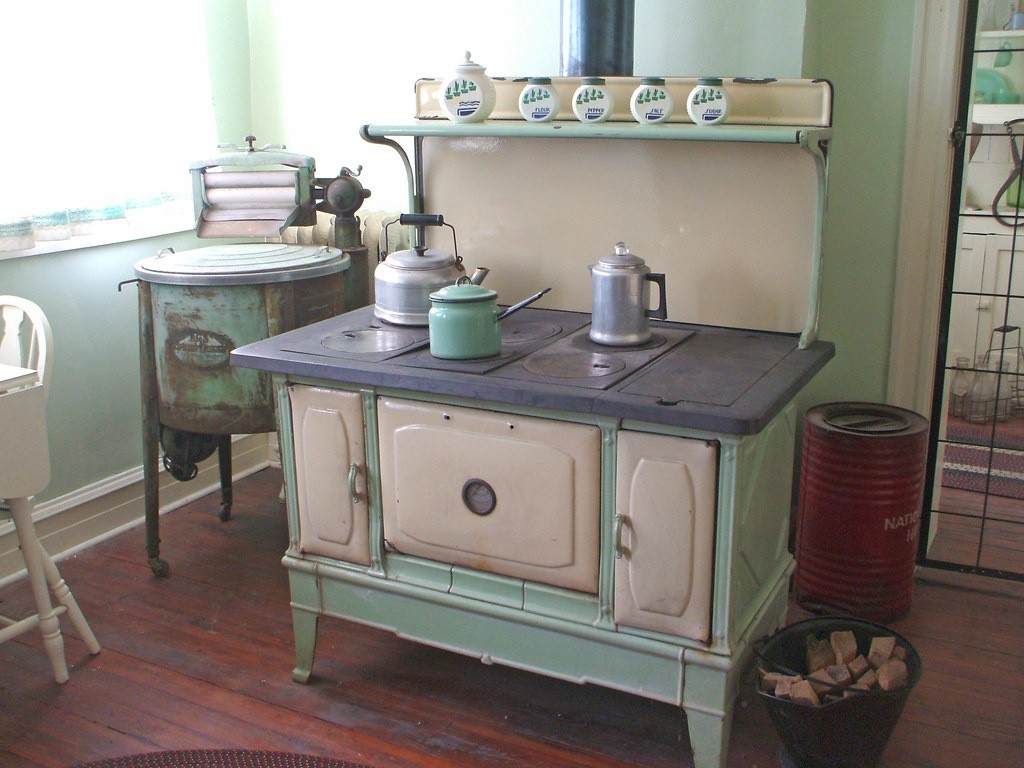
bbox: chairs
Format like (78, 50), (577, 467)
(1, 294), (102, 687)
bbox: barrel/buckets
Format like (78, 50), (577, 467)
(794, 402), (930, 624)
(752, 615), (921, 768)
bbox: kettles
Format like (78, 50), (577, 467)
(588, 243), (667, 345)
(374, 214), (490, 325)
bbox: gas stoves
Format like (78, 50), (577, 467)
(229, 303), (834, 434)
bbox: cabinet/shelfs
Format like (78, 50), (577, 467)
(269, 370), (797, 768)
(942, 214), (1024, 409)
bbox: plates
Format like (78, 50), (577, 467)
(974, 69), (1009, 104)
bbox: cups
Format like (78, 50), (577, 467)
(992, 94), (1021, 104)
(993, 41), (1013, 67)
(1006, 177), (1024, 208)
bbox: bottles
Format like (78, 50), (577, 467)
(572, 79), (613, 124)
(440, 50), (496, 123)
(518, 77), (559, 123)
(687, 79), (732, 125)
(630, 79), (673, 124)
(949, 355), (1011, 424)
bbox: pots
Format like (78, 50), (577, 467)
(428, 276), (551, 358)
